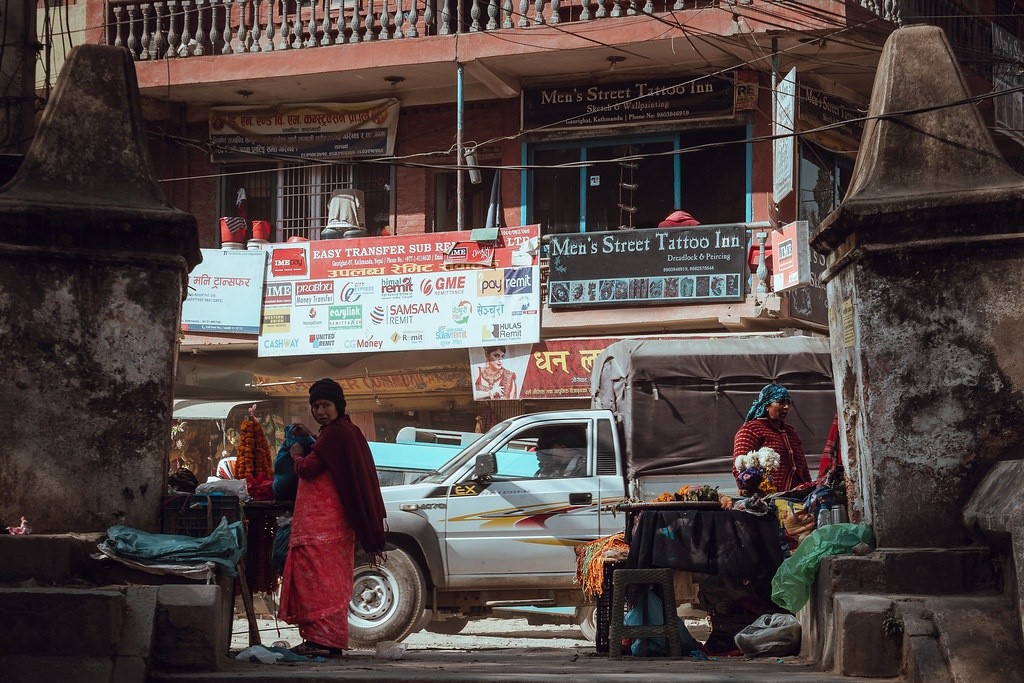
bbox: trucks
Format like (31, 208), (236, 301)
(342, 335), (838, 643)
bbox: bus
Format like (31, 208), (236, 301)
(342, 427), (539, 487)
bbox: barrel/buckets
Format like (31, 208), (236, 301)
(219, 218), (246, 243)
(287, 236), (308, 242)
(252, 220), (271, 240)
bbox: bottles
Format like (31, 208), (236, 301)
(270, 641), (291, 649)
(817, 504), (832, 529)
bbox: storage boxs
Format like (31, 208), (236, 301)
(179, 494), (238, 539)
(596, 560), (626, 653)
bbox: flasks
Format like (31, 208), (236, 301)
(831, 498), (848, 524)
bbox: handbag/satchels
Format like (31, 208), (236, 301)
(272, 423), (317, 500)
(734, 613), (803, 656)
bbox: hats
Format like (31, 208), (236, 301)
(310, 379), (346, 415)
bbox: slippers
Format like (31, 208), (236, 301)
(329, 648), (343, 655)
(288, 639), (329, 656)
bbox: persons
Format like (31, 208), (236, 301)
(276, 378), (357, 659)
(533, 428), (586, 477)
(732, 384), (811, 501)
(471, 346), (517, 400)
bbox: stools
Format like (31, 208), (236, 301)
(609, 568), (681, 657)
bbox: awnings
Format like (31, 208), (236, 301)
(171, 399), (274, 419)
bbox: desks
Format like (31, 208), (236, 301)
(622, 510), (788, 657)
